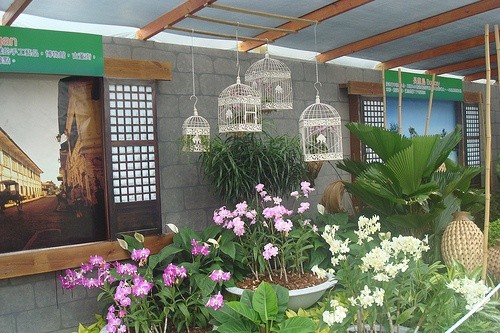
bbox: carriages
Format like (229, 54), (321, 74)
(0, 180), (24, 216)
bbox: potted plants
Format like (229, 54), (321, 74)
(182, 11), (347, 203)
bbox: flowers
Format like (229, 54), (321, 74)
(57, 180), (499, 333)
